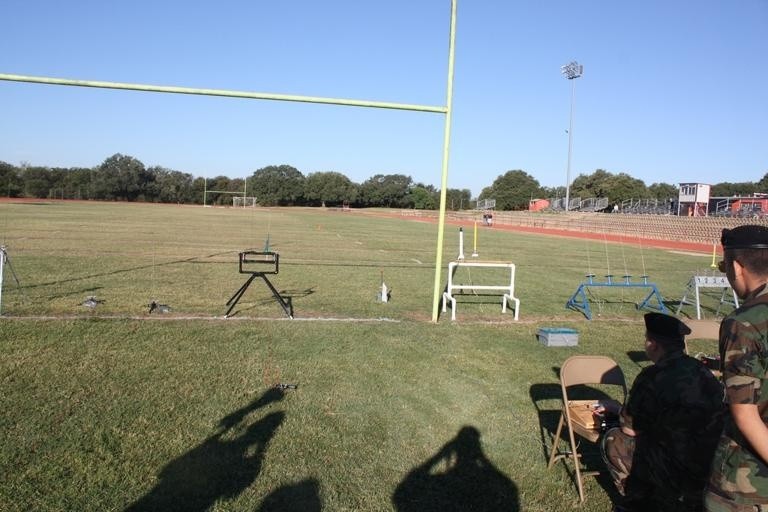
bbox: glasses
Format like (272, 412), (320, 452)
(717, 261), (728, 275)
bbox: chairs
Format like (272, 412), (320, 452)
(547, 351), (629, 500)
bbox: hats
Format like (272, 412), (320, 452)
(721, 225), (768, 250)
(643, 312), (692, 347)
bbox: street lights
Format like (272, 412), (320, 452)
(560, 59), (584, 213)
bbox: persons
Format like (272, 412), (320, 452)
(624, 313), (723, 512)
(703, 226), (768, 512)
